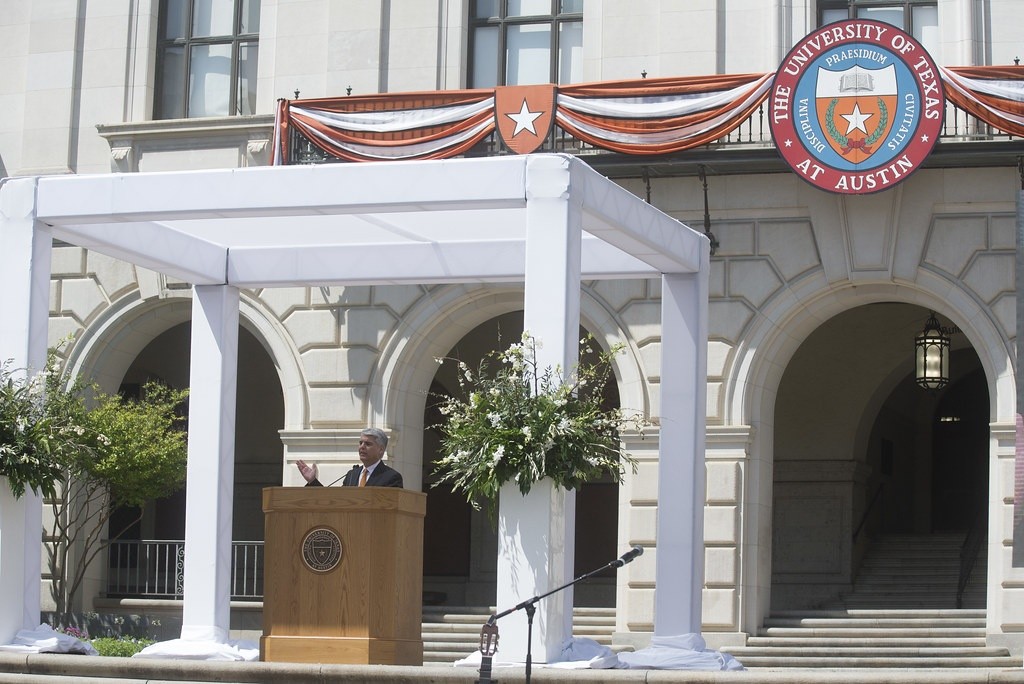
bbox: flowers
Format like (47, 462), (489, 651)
(0, 332), (112, 502)
(417, 320), (662, 534)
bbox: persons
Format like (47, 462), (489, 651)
(296, 428), (403, 487)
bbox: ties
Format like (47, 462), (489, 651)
(359, 469), (368, 487)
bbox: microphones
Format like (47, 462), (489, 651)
(613, 546), (644, 568)
(327, 465), (359, 487)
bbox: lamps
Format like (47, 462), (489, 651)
(938, 416), (961, 425)
(912, 310), (951, 393)
